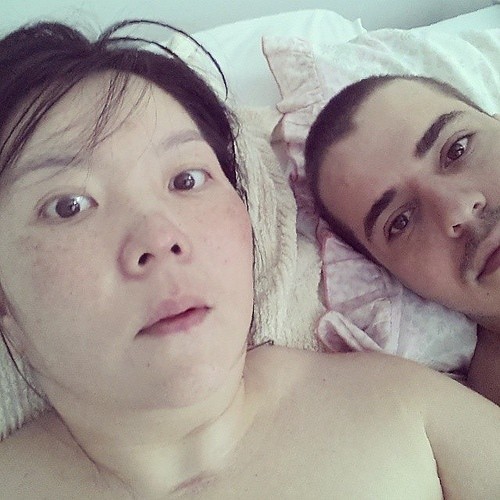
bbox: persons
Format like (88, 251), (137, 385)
(1, 9), (500, 500)
(303, 73), (500, 407)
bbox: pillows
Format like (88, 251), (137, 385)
(0, 11), (367, 445)
(262, 3), (500, 373)
